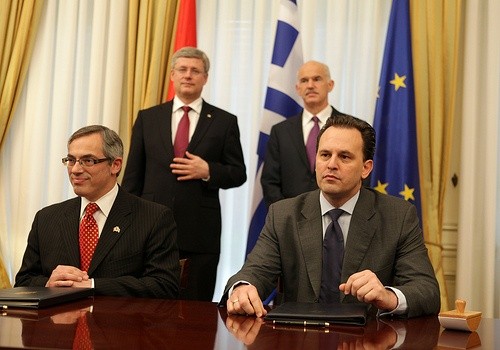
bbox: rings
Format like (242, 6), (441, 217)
(232, 326), (238, 333)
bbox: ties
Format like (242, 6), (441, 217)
(173, 106), (192, 158)
(305, 116), (320, 174)
(79, 203), (100, 272)
(319, 209), (344, 303)
(73, 312), (94, 350)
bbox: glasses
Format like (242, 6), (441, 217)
(172, 67), (205, 75)
(62, 157), (111, 166)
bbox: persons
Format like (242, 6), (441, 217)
(14, 125), (181, 301)
(218, 306), (441, 350)
(19, 300), (183, 350)
(259, 60), (342, 204)
(215, 112), (440, 317)
(120, 47), (248, 301)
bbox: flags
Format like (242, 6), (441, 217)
(366, 0), (423, 237)
(165, 0), (197, 103)
(242, 0), (304, 307)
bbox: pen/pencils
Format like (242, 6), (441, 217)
(272, 320), (330, 327)
(0, 305), (8, 309)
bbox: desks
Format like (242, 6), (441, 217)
(1, 290), (500, 350)
